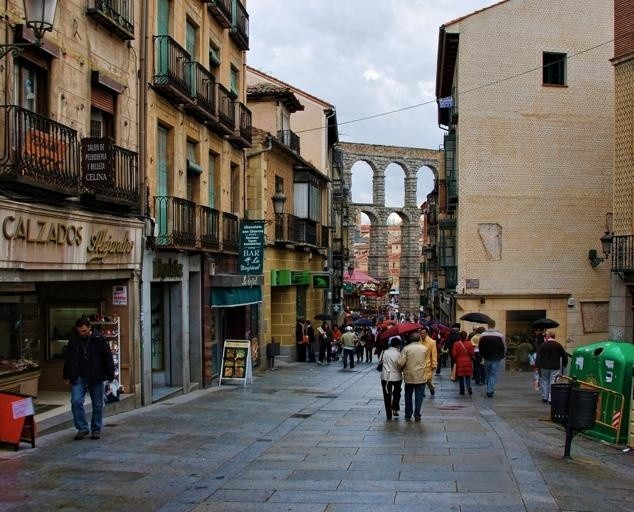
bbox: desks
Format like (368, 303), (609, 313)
(0, 366), (43, 396)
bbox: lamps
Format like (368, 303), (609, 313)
(264, 189), (286, 227)
(480, 297), (485, 304)
(589, 231), (612, 267)
(425, 242), (441, 271)
(0, 0), (57, 61)
(343, 265), (354, 280)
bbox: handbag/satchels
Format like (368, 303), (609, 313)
(377, 362), (383, 371)
(450, 363), (457, 381)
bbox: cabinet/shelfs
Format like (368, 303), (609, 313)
(89, 317), (121, 384)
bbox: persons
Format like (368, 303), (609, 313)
(296, 295), (506, 422)
(62, 318), (115, 440)
(515, 327), (568, 404)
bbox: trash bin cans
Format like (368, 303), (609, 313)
(550, 341), (634, 461)
(267, 336), (280, 368)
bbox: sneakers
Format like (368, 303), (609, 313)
(75, 432), (89, 440)
(431, 389), (434, 395)
(487, 392), (494, 397)
(387, 409), (420, 421)
(543, 399), (551, 404)
(92, 430), (99, 439)
(460, 388), (472, 395)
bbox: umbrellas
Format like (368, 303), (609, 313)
(530, 318), (560, 336)
